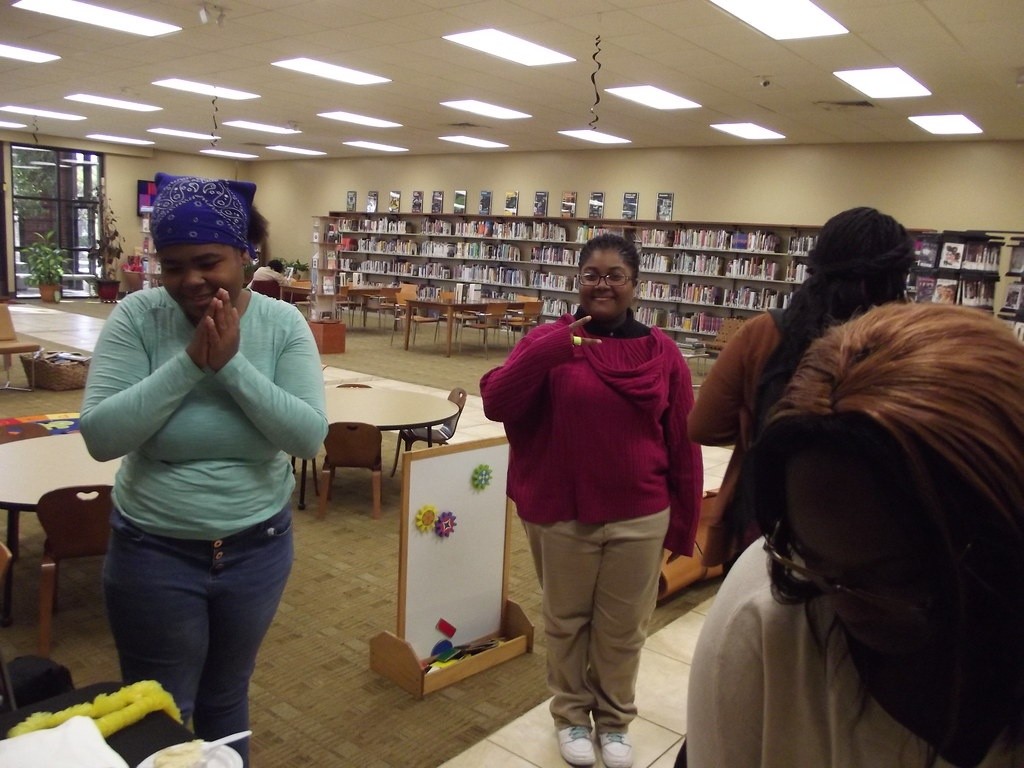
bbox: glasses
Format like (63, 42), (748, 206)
(761, 514), (949, 631)
(574, 272), (635, 287)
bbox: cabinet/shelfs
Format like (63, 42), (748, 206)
(309, 216), (345, 354)
(330, 211), (1005, 342)
(997, 238), (1024, 322)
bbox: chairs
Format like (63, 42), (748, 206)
(36, 485), (113, 661)
(697, 318), (752, 375)
(391, 388), (466, 477)
(253, 277), (544, 361)
(0, 304), (40, 392)
(318, 423), (383, 520)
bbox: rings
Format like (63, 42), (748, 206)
(573, 337), (582, 346)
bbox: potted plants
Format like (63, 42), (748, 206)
(87, 186), (128, 303)
(20, 231), (75, 303)
(273, 257), (309, 280)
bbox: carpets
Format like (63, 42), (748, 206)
(0, 412), (94, 445)
(0, 332), (724, 768)
(12, 298), (736, 451)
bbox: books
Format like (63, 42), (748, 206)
(322, 191), (1024, 355)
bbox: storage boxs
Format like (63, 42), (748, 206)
(20, 354), (90, 391)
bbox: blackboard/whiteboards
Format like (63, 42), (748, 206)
(397, 437), (513, 665)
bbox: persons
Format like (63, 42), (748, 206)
(80, 173), (329, 768)
(686, 206), (915, 582)
(688, 299), (1024, 768)
(479, 231), (702, 768)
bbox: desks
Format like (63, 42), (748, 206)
(0, 433), (126, 622)
(291, 375), (459, 510)
(0, 680), (193, 768)
(682, 353), (709, 379)
(404, 296), (526, 358)
(281, 282), (381, 327)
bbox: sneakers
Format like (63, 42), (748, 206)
(557, 725), (596, 766)
(599, 731), (631, 768)
